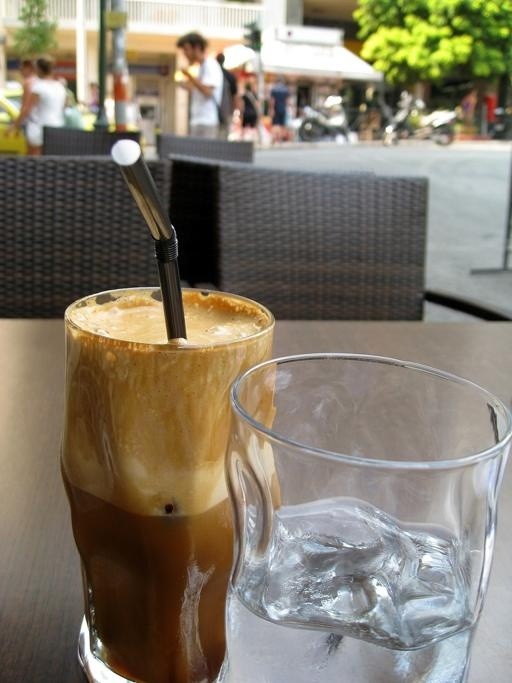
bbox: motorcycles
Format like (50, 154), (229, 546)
(383, 90), (458, 148)
(297, 98), (349, 142)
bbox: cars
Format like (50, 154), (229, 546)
(0, 83), (149, 160)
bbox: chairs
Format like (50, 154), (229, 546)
(1, 125), (428, 320)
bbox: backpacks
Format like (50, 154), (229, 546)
(221, 67), (238, 95)
(210, 74), (233, 128)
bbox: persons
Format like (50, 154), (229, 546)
(174, 32), (223, 138)
(239, 83), (262, 145)
(0, 54), (99, 158)
(215, 53), (237, 140)
(269, 79), (289, 144)
(462, 90), (496, 133)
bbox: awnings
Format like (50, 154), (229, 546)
(243, 38), (382, 80)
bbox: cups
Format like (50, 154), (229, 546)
(228, 352), (511, 683)
(61, 288), (279, 683)
